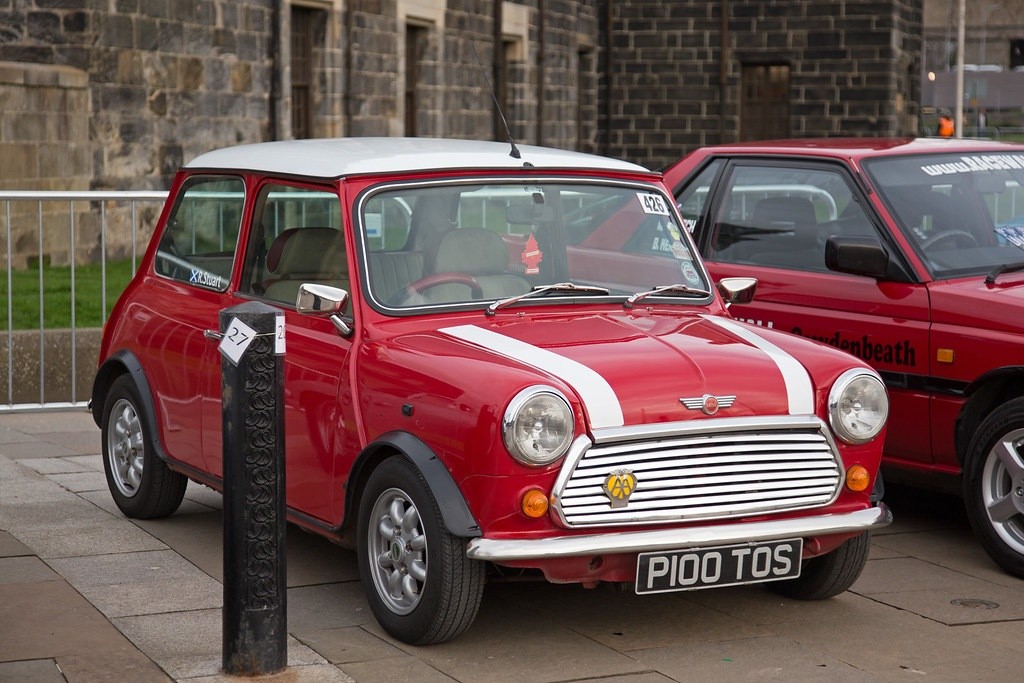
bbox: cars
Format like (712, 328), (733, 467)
(493, 133), (1024, 578)
(86, 135), (890, 649)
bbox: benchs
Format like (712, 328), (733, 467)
(190, 248), (428, 319)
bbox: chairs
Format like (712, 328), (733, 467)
(725, 197), (824, 273)
(422, 226), (529, 304)
(256, 228), (366, 318)
